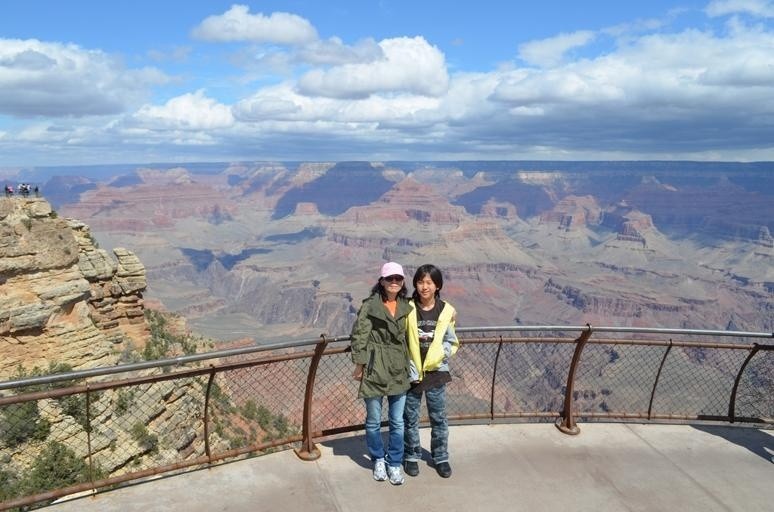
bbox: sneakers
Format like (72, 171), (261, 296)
(373, 457), (388, 481)
(387, 464), (405, 485)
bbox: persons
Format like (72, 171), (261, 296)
(347, 260), (458, 487)
(402, 264), (460, 479)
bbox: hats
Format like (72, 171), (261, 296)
(380, 261), (406, 278)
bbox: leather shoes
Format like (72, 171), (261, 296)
(435, 462), (452, 478)
(404, 461), (419, 476)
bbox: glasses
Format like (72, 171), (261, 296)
(382, 276), (403, 282)
